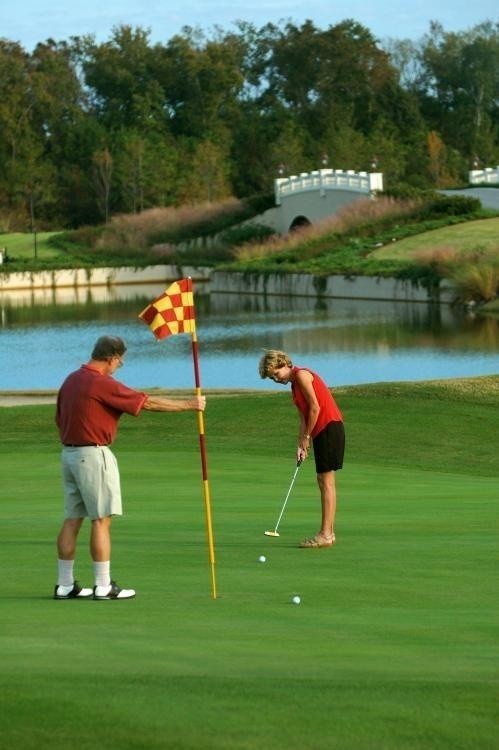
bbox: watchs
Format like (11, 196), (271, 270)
(303, 434), (311, 440)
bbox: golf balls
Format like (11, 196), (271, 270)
(259, 555), (266, 563)
(292, 596), (301, 605)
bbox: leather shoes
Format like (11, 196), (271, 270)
(94, 581), (136, 600)
(54, 580), (94, 599)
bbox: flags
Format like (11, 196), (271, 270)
(137, 279), (196, 340)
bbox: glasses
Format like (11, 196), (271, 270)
(115, 356), (124, 368)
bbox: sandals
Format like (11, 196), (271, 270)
(297, 532), (336, 549)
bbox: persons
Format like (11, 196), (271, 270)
(258, 347), (346, 548)
(54, 332), (207, 601)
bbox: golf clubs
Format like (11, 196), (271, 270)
(264, 454), (303, 537)
